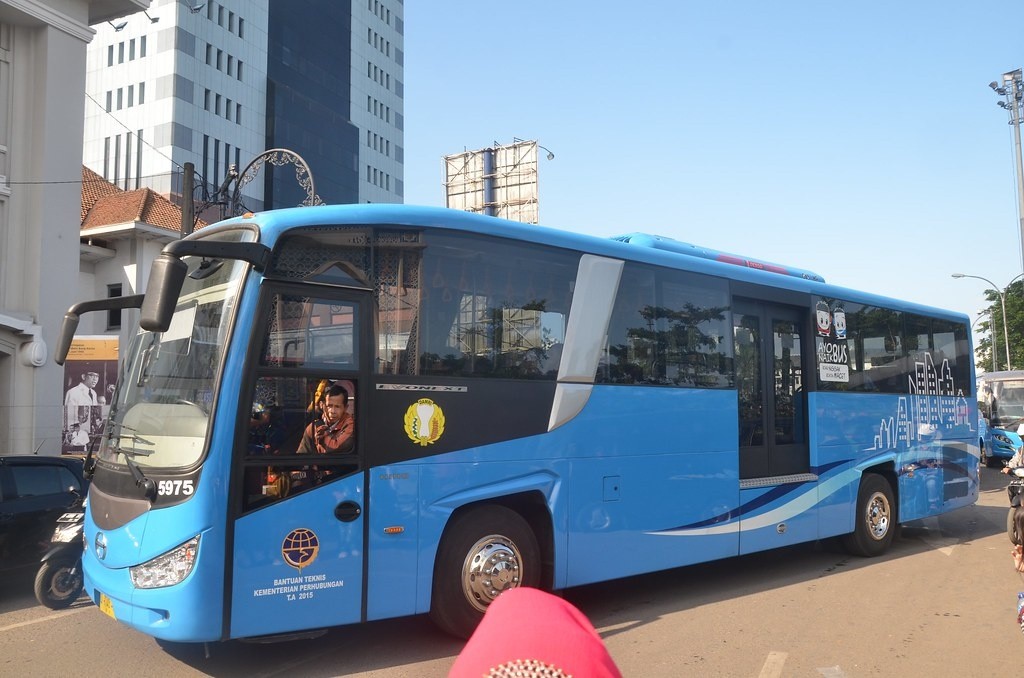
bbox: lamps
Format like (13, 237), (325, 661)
(108, 20), (127, 32)
(185, 0), (205, 14)
(143, 9), (159, 24)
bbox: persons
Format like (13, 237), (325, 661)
(98, 396), (106, 405)
(1001, 424), (1024, 503)
(291, 385), (354, 493)
(62, 424), (90, 451)
(521, 348), (553, 380)
(902, 423), (943, 509)
(64, 366), (100, 405)
(92, 416), (105, 435)
(1011, 508), (1024, 633)
(307, 378), (333, 413)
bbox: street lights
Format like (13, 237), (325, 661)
(988, 67), (1024, 290)
(949, 272), (1024, 371)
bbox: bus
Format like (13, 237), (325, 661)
(977, 370), (1024, 467)
(52, 201), (981, 646)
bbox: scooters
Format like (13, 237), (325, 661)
(34, 485), (91, 612)
(999, 460), (1024, 546)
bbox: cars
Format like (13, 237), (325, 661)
(1, 454), (88, 597)
